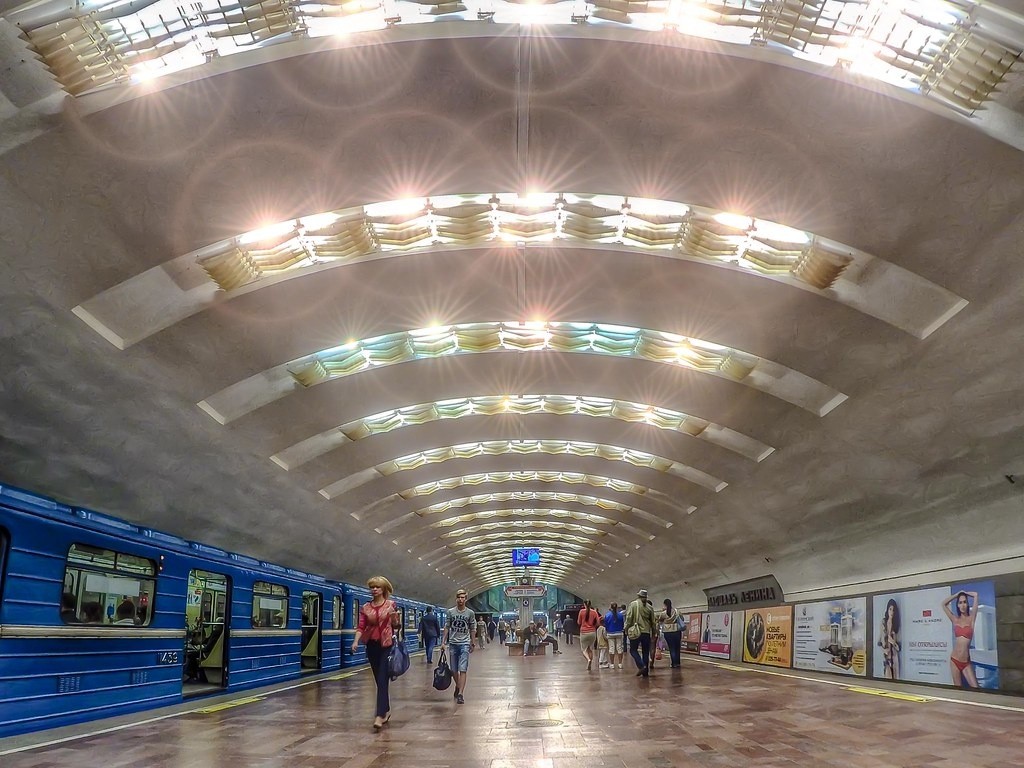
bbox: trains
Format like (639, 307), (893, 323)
(0, 485), (449, 737)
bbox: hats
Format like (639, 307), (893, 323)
(637, 590), (648, 597)
(529, 620), (534, 624)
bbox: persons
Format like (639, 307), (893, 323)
(61, 593), (150, 626)
(876, 599), (901, 679)
(478, 615), (520, 650)
(185, 615), (340, 682)
(702, 615), (712, 643)
(941, 590), (980, 688)
(417, 606), (440, 664)
(522, 620), (562, 656)
(746, 617), (764, 657)
(441, 589), (477, 704)
(577, 589), (685, 677)
(520, 548), (540, 561)
(551, 614), (575, 644)
(351, 576), (401, 734)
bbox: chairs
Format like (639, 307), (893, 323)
(301, 629), (318, 668)
(201, 631), (223, 683)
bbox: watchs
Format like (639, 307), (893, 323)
(471, 643), (476, 647)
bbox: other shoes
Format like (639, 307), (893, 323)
(619, 663), (622, 669)
(457, 693), (464, 704)
(635, 666), (647, 676)
(600, 663), (610, 669)
(611, 664), (615, 669)
(587, 659), (591, 671)
(524, 653), (527, 656)
(650, 661), (654, 669)
(454, 685), (459, 698)
(669, 662), (680, 667)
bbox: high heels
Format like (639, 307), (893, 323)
(374, 717), (383, 733)
(382, 713), (390, 724)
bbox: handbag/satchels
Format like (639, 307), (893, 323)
(674, 609), (686, 631)
(627, 623), (640, 640)
(655, 647), (662, 660)
(433, 650), (452, 690)
(387, 624), (410, 680)
(487, 637), (490, 643)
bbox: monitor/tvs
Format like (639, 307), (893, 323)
(512, 548), (540, 566)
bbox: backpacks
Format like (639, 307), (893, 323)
(490, 621), (495, 630)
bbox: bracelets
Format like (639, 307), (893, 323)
(442, 642), (446, 645)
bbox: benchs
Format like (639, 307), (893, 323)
(505, 641), (549, 656)
